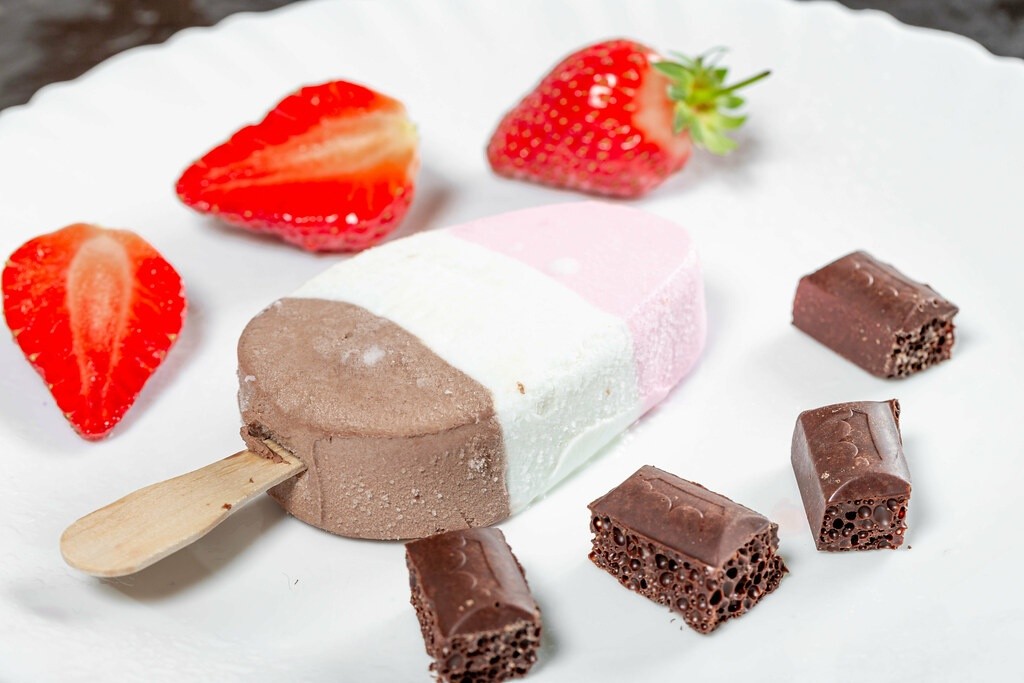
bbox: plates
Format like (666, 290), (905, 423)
(0, 1), (1024, 683)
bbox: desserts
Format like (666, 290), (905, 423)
(402, 248), (962, 683)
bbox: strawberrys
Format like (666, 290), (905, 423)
(482, 38), (771, 204)
(1, 221), (190, 442)
(174, 80), (419, 255)
